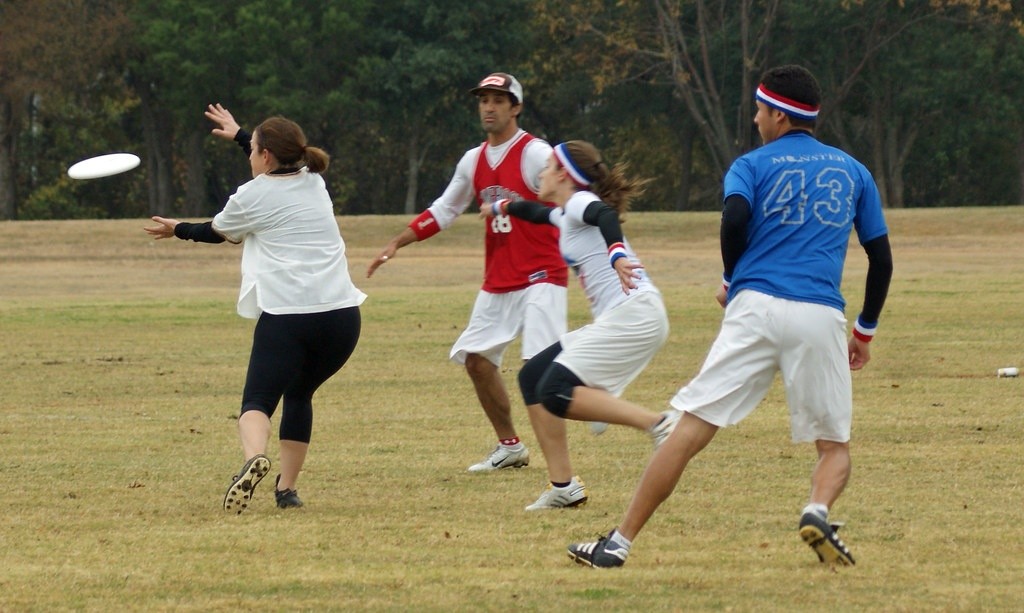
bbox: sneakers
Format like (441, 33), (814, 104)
(221, 454), (272, 515)
(592, 421), (609, 435)
(274, 473), (302, 510)
(799, 507), (855, 568)
(468, 443), (531, 471)
(651, 410), (683, 449)
(525, 476), (588, 511)
(567, 533), (630, 570)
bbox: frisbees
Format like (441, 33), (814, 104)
(67, 153), (141, 179)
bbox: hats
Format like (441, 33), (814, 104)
(470, 72), (523, 104)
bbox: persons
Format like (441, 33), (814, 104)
(365, 73), (609, 471)
(479, 140), (684, 510)
(143, 102), (369, 516)
(569, 65), (893, 570)
